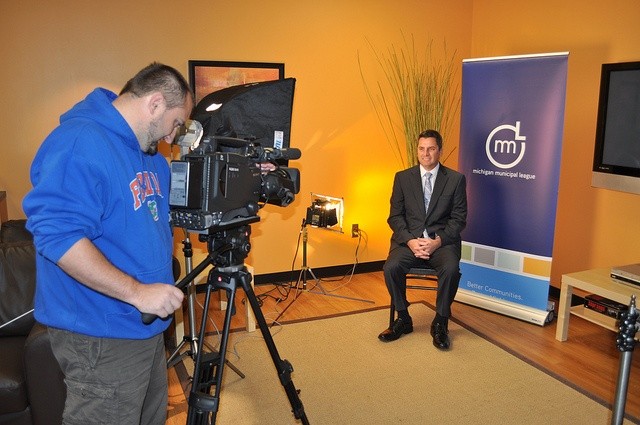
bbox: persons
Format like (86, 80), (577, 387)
(378, 130), (467, 351)
(22, 63), (195, 425)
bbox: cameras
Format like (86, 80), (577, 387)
(164, 134), (299, 231)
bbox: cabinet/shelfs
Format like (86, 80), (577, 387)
(1, 190), (7, 222)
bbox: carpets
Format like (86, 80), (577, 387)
(166, 297), (640, 425)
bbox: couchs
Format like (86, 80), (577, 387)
(0, 223), (67, 424)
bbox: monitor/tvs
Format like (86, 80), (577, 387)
(589, 60), (639, 194)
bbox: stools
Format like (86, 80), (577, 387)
(387, 262), (449, 330)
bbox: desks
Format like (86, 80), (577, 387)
(555, 265), (639, 342)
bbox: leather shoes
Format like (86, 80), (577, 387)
(378, 315), (414, 342)
(430, 320), (450, 349)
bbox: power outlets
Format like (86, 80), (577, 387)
(352, 224), (358, 238)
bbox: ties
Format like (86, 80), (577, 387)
(422, 172), (432, 239)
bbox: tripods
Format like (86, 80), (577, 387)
(271, 227), (374, 326)
(165, 228), (245, 380)
(143, 227), (311, 425)
